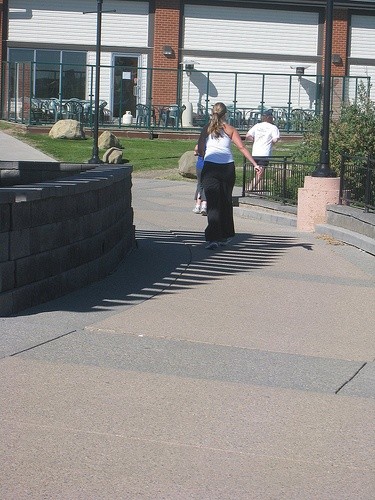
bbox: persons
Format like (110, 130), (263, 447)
(198, 102), (263, 250)
(245, 112), (282, 195)
(193, 125), (208, 216)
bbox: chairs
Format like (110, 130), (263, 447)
(190, 103), (315, 131)
(135, 104), (186, 128)
(31, 98), (113, 123)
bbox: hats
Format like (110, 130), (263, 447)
(262, 112), (275, 118)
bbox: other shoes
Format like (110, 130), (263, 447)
(220, 238), (232, 246)
(205, 241), (218, 248)
(201, 207), (208, 216)
(192, 207), (200, 213)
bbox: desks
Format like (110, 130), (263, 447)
(60, 100), (89, 106)
(271, 106), (290, 108)
(231, 108), (258, 128)
(149, 105), (178, 125)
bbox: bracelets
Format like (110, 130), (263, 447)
(254, 165), (259, 168)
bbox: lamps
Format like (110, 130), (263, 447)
(332, 54), (341, 65)
(180, 59), (200, 75)
(163, 46), (173, 54)
(291, 64), (310, 79)
